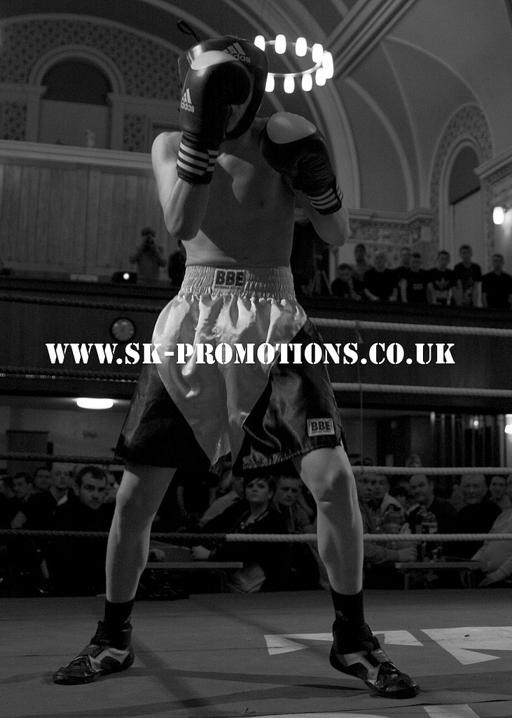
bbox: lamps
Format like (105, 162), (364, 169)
(253, 31), (337, 94)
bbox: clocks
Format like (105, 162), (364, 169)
(107, 315), (138, 344)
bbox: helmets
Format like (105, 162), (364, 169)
(175, 32), (268, 140)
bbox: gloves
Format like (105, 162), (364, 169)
(173, 52), (256, 186)
(257, 109), (345, 216)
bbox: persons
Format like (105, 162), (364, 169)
(125, 221), (512, 316)
(54, 37), (419, 701)
(0, 451), (511, 596)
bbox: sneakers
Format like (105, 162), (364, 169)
(327, 616), (423, 701)
(54, 622), (137, 686)
